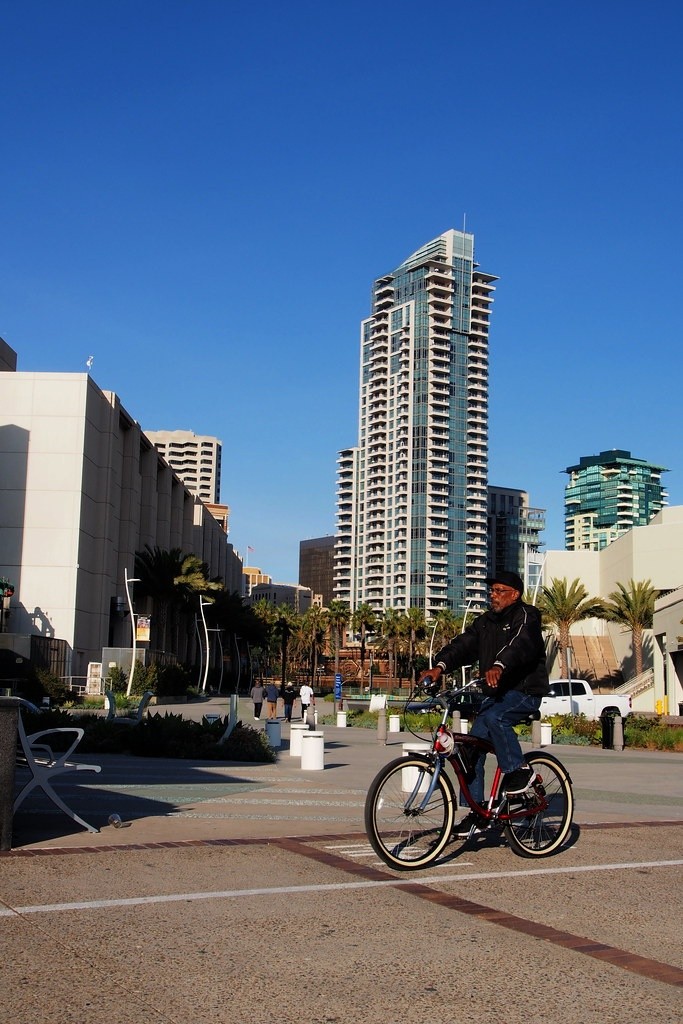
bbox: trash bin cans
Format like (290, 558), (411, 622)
(598, 705), (625, 752)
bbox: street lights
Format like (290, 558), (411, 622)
(199, 595), (214, 691)
(123, 567), (143, 700)
(196, 614), (204, 688)
(233, 632), (244, 694)
(216, 624), (226, 695)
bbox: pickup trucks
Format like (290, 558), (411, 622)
(533, 680), (633, 720)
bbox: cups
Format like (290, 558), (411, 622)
(108, 813), (122, 828)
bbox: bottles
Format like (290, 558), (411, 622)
(453, 742), (473, 776)
(435, 732), (458, 755)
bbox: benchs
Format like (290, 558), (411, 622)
(0, 695), (105, 835)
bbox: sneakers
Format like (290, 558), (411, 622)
(505, 761), (536, 794)
(452, 801), (491, 836)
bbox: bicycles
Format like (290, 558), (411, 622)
(364, 676), (576, 872)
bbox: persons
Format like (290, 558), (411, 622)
(418, 572), (549, 839)
(250, 680), (315, 724)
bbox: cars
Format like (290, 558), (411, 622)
(406, 688), (485, 718)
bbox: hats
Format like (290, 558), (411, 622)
(484, 571), (524, 594)
(288, 682), (292, 686)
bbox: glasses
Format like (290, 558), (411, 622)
(489, 587), (515, 594)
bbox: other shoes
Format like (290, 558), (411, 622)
(254, 717), (260, 721)
(285, 718), (288, 723)
